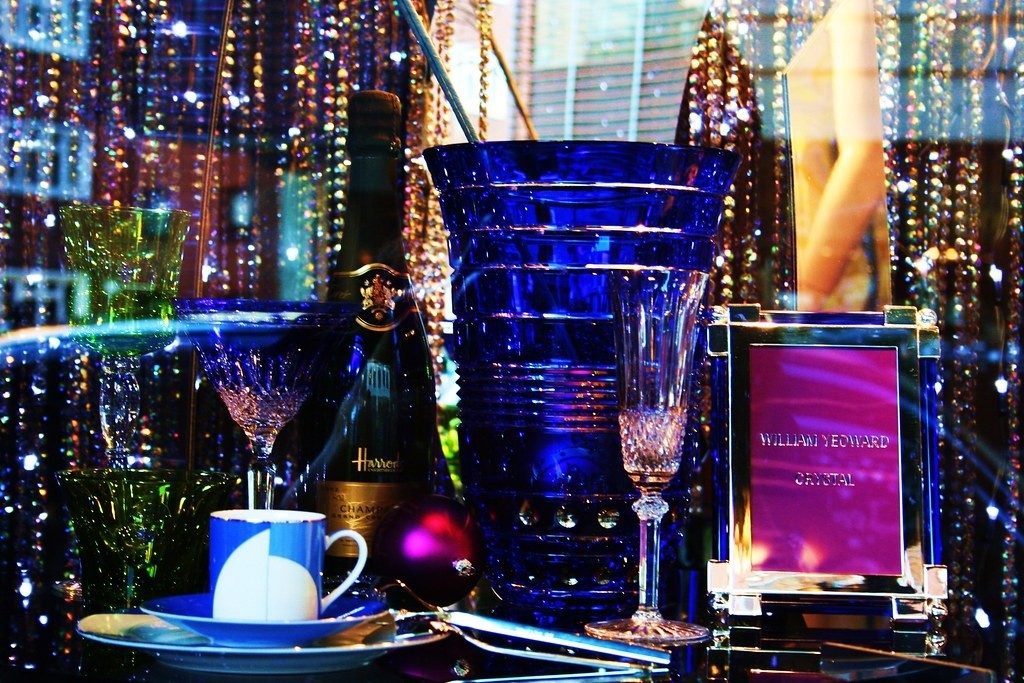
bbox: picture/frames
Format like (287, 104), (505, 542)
(708, 305), (946, 629)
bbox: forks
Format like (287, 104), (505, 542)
(393, 578), (671, 665)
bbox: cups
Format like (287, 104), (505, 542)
(421, 139), (742, 638)
(210, 509), (368, 623)
(57, 463), (237, 616)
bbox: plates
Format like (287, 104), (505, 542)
(139, 593), (390, 648)
(74, 608), (451, 674)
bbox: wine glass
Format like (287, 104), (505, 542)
(170, 296), (361, 514)
(54, 204), (193, 467)
(584, 264), (712, 640)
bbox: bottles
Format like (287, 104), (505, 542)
(307, 91), (439, 595)
